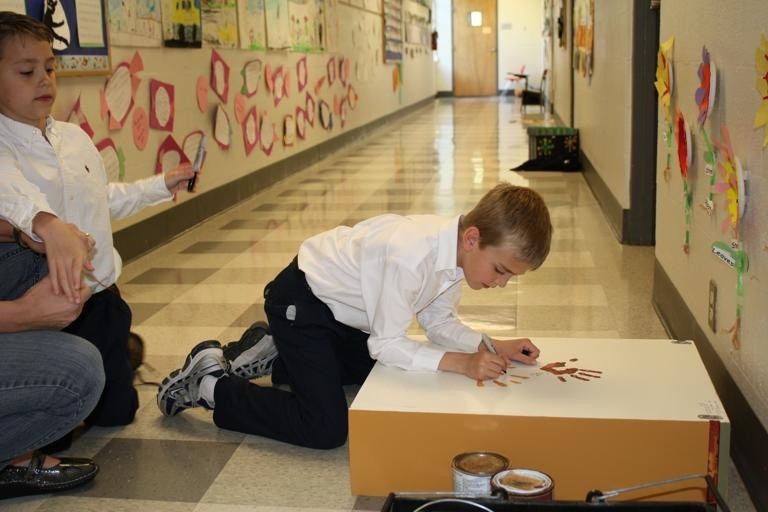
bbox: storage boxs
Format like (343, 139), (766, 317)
(527, 126), (579, 160)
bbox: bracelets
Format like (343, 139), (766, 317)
(11, 228), (27, 249)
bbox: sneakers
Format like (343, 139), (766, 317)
(156, 340), (229, 417)
(225, 321), (280, 380)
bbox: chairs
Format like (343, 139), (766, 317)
(500, 65), (526, 96)
(521, 69), (547, 114)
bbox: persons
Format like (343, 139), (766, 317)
(156, 182), (553, 448)
(2, 11), (200, 426)
(1, 205), (106, 496)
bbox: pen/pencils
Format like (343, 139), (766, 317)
(482, 333), (507, 374)
(187, 135), (208, 192)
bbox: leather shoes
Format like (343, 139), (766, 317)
(0, 449), (99, 500)
